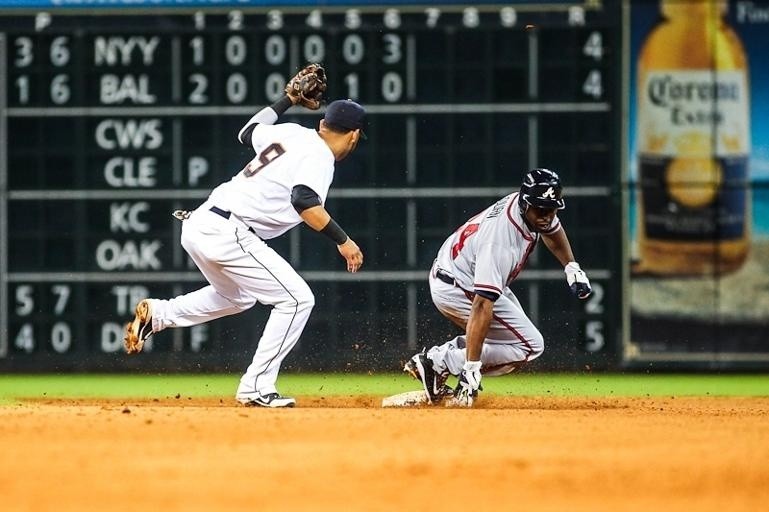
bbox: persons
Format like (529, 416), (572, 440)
(124, 62), (368, 407)
(400, 168), (593, 406)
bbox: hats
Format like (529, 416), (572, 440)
(325, 98), (368, 140)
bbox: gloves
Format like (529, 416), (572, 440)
(564, 262), (592, 300)
(453, 361), (483, 401)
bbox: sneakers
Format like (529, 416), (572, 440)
(404, 347), (453, 406)
(124, 300), (153, 354)
(251, 394), (296, 407)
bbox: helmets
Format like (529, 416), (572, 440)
(518, 168), (566, 214)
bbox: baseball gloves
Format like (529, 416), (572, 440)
(284, 63), (327, 110)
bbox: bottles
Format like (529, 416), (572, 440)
(633, 0), (752, 284)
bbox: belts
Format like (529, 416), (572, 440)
(210, 206), (256, 234)
(436, 272), (454, 285)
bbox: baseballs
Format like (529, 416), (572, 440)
(462, 397), (473, 407)
